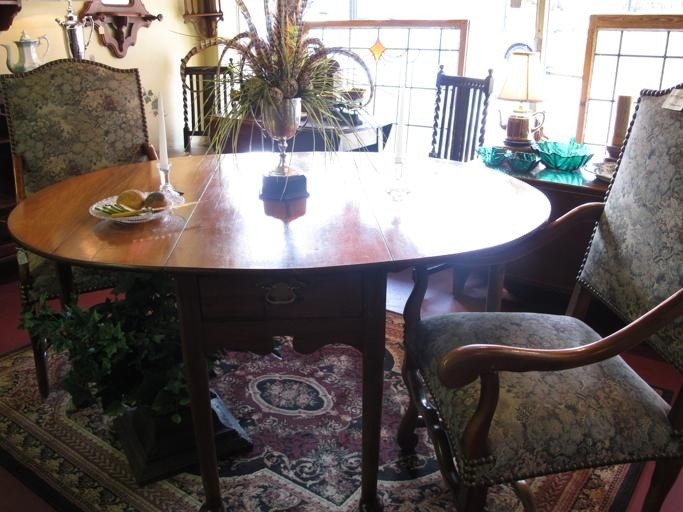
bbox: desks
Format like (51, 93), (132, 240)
(205, 114), (392, 154)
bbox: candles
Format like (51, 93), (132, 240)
(612, 95), (631, 147)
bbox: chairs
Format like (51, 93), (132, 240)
(0, 58), (158, 400)
(397, 84), (683, 512)
(180, 57), (235, 151)
(427, 65), (493, 163)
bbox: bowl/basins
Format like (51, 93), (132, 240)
(503, 152), (542, 174)
(477, 146), (507, 168)
(531, 141), (594, 171)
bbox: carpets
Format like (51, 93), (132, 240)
(0, 310), (675, 512)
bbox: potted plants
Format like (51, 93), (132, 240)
(176, 0), (386, 202)
(17, 273), (254, 488)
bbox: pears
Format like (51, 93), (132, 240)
(145, 192), (167, 208)
(116, 189), (146, 210)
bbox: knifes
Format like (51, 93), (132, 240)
(109, 200), (200, 218)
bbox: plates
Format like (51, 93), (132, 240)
(504, 142), (532, 147)
(89, 191), (185, 225)
(584, 161), (615, 182)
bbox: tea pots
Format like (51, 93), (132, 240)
(1, 31), (50, 74)
(54, 2), (95, 59)
(497, 106), (546, 142)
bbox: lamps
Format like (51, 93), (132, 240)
(494, 51), (546, 153)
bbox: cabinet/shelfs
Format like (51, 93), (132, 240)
(500, 177), (605, 294)
